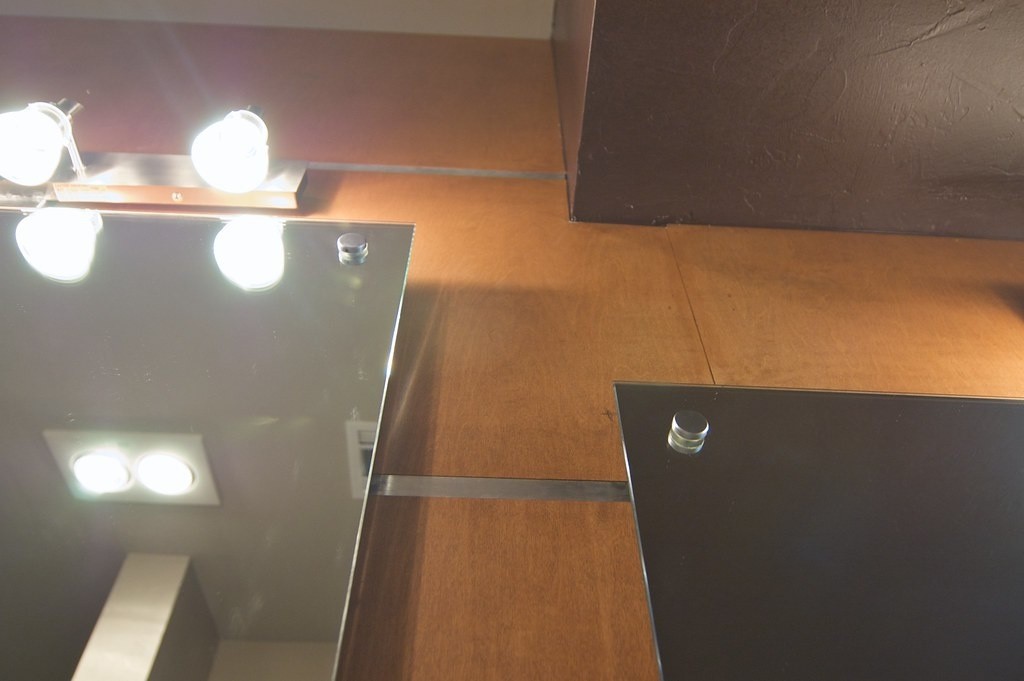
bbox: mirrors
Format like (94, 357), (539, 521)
(0, 211), (417, 681)
(611, 378), (1023, 681)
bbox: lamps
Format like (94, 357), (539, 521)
(44, 430), (221, 507)
(0, 95), (90, 187)
(14, 206), (104, 284)
(213, 214), (288, 291)
(191, 103), (271, 194)
